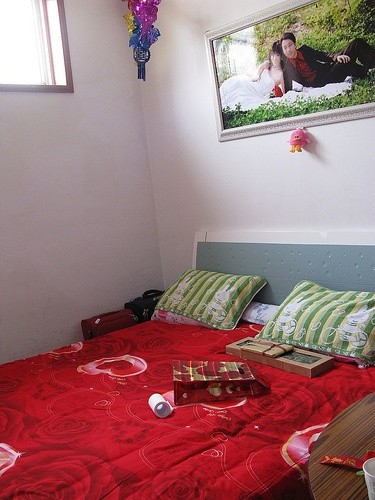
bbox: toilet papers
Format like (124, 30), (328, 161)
(147, 393), (174, 418)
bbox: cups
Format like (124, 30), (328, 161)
(362, 458), (375, 500)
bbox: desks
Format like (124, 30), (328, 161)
(310, 395), (375, 500)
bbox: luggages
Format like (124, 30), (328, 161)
(126, 290), (166, 323)
(81, 308), (135, 341)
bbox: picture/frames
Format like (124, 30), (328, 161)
(202, 0), (375, 142)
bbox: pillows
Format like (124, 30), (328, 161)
(155, 269), (265, 331)
(254, 279), (375, 366)
(152, 310), (214, 330)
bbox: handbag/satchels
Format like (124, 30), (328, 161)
(172, 362), (270, 405)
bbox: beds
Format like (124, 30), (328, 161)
(0, 231), (375, 500)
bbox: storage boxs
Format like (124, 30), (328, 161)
(226, 337), (335, 378)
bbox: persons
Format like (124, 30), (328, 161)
(219, 32), (375, 113)
